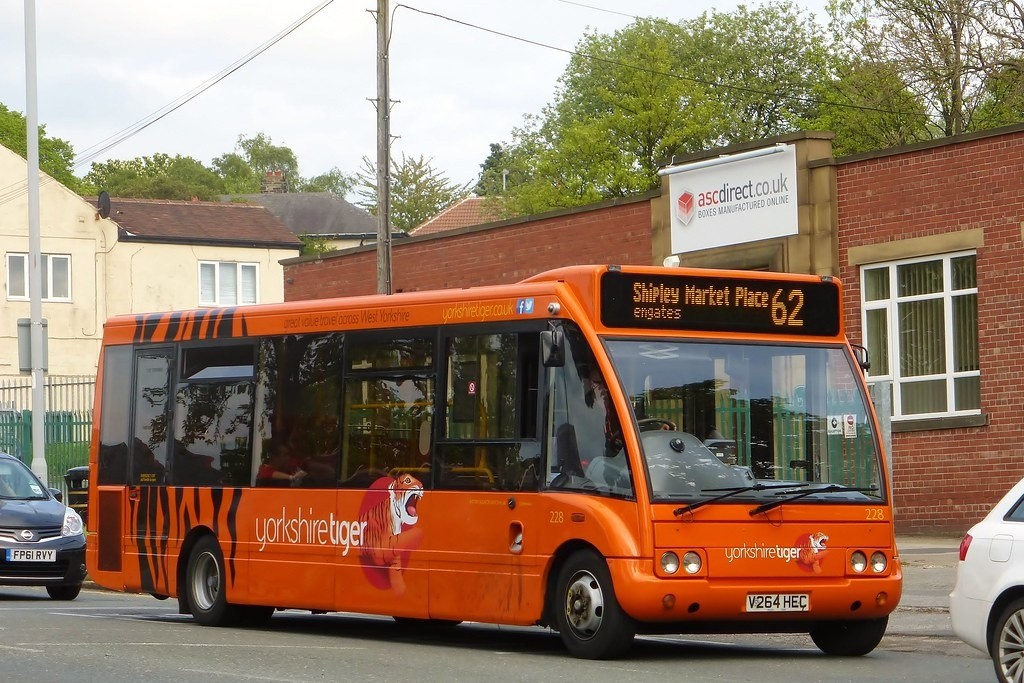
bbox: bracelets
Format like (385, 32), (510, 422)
(289, 475), (293, 481)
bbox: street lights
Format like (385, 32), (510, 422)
(502, 168), (509, 191)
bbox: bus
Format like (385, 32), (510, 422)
(86, 263), (904, 656)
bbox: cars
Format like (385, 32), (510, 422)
(0, 450), (85, 601)
(947, 477), (1024, 683)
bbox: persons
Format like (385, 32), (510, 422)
(256, 444), (309, 482)
(576, 366), (677, 459)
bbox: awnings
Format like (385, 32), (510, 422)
(187, 365), (253, 380)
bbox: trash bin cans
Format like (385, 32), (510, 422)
(63, 465), (90, 533)
